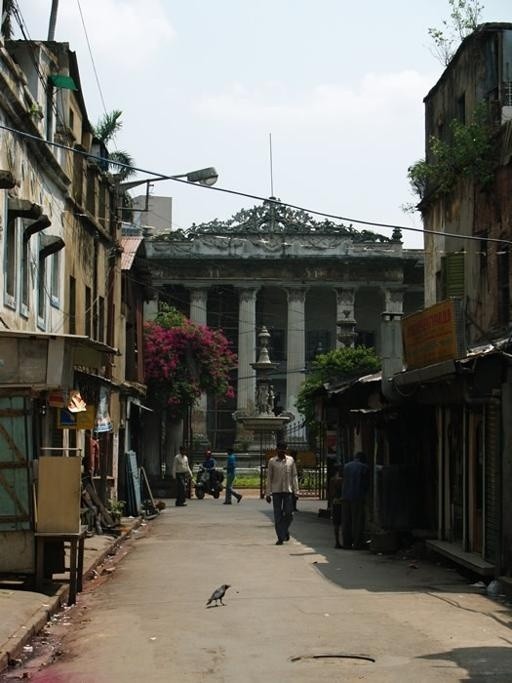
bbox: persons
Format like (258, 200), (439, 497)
(223, 449), (243, 504)
(329, 463), (343, 548)
(171, 446), (193, 506)
(265, 440), (299, 545)
(288, 449), (298, 512)
(340, 451), (372, 549)
(202, 450), (217, 492)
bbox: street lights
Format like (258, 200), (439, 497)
(248, 323), (283, 410)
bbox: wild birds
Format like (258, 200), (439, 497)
(207, 583), (231, 607)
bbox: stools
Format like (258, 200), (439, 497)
(34, 524), (88, 605)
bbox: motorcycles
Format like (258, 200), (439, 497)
(194, 462), (224, 498)
